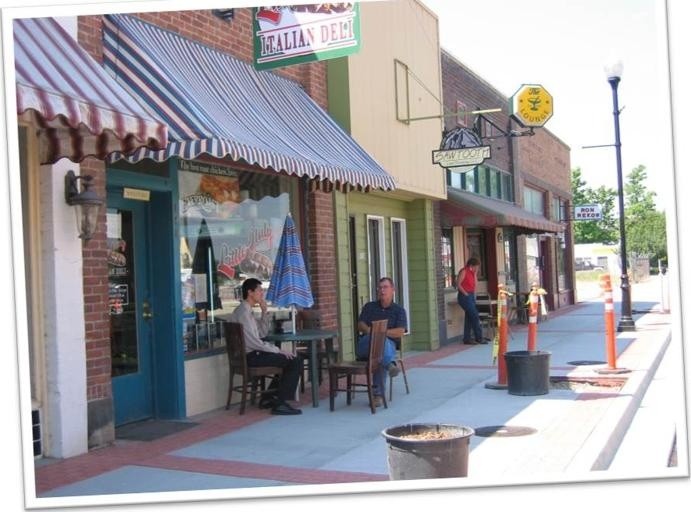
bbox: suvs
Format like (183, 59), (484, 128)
(573, 258), (604, 271)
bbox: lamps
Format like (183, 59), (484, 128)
(64, 170), (106, 243)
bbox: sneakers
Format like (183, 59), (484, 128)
(370, 396), (384, 407)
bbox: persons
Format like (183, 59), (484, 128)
(355, 277), (409, 407)
(457, 257), (490, 345)
(226, 277), (305, 415)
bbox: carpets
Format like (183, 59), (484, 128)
(114, 417), (202, 442)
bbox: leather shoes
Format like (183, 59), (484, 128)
(258, 396), (302, 415)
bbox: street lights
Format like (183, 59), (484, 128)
(580, 57), (638, 334)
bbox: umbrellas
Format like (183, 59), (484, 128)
(264, 210), (316, 402)
(191, 217), (224, 312)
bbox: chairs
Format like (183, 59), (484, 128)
(223, 322), (291, 415)
(296, 313), (340, 395)
(327, 318), (390, 413)
(448, 290), (545, 341)
(355, 330), (411, 402)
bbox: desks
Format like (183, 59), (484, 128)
(262, 329), (342, 407)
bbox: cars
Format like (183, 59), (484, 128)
(235, 272), (272, 299)
